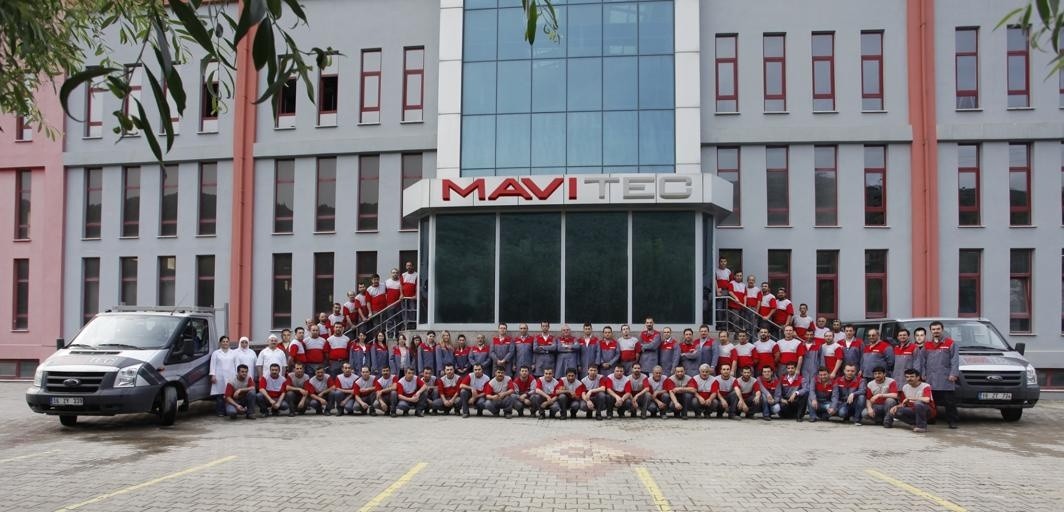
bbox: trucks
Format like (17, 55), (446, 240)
(831, 317), (1039, 427)
(21, 296), (234, 429)
